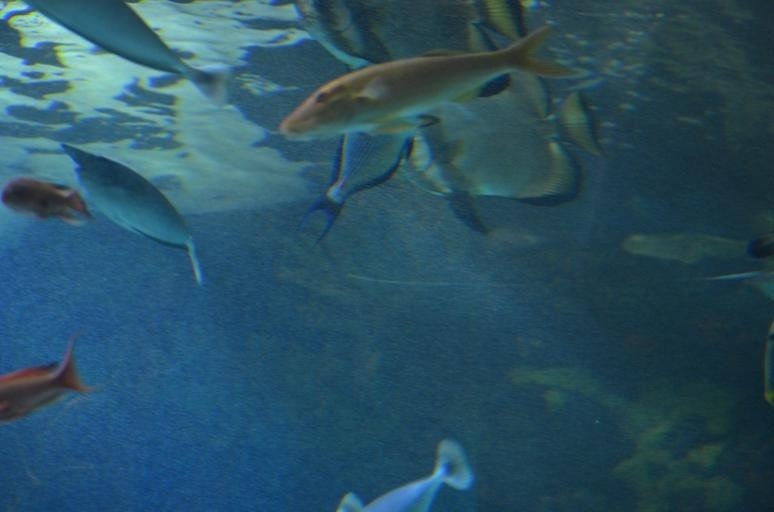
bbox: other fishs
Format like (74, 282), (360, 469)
(1, 177), (93, 227)
(76, 156), (214, 291)
(0, 335), (98, 422)
(25, 0), (232, 106)
(278, 1), (609, 246)
(692, 271), (774, 299)
(336, 439), (473, 512)
(625, 232), (774, 267)
(763, 322), (774, 408)
